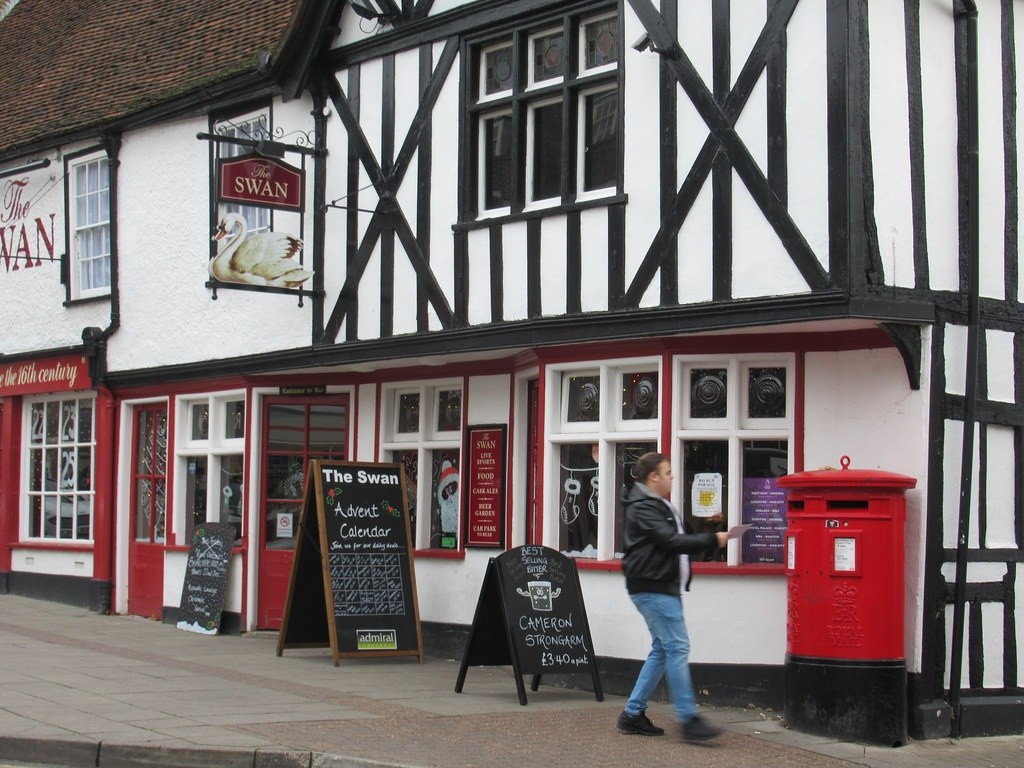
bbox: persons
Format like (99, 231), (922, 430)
(616, 451), (728, 743)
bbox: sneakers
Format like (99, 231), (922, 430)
(617, 712), (663, 735)
(682, 717), (726, 742)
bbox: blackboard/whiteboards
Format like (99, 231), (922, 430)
(176, 522), (236, 635)
(495, 544), (599, 676)
(314, 460), (425, 658)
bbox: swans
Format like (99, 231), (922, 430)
(209, 212), (316, 289)
